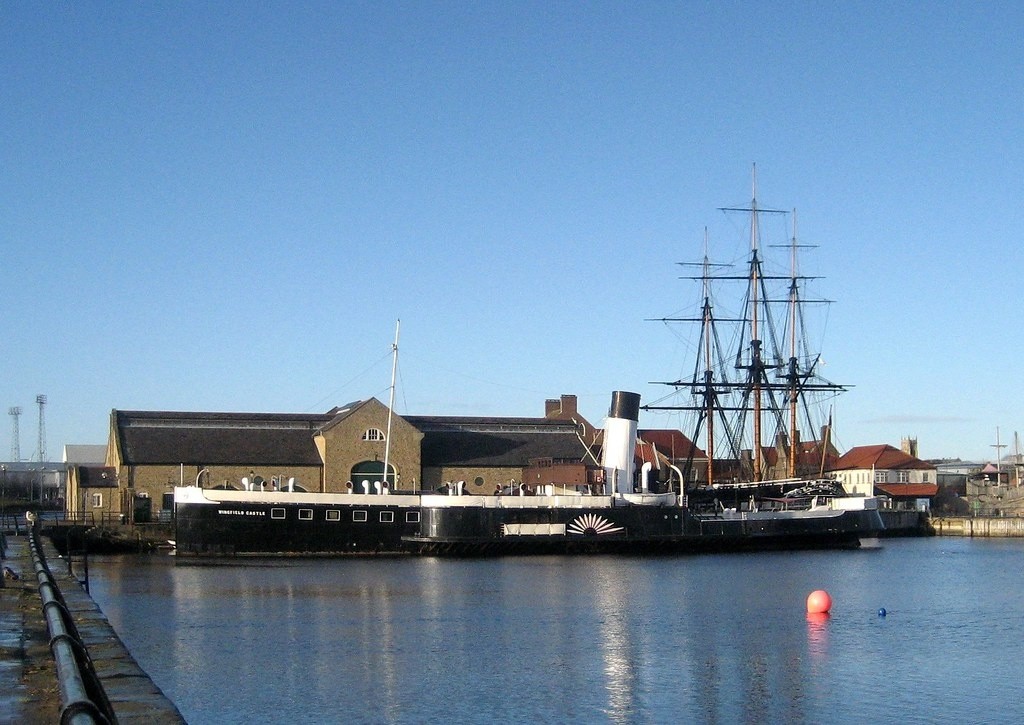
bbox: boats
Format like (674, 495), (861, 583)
(174, 315), (689, 559)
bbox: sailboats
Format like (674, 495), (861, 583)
(639, 160), (885, 554)
